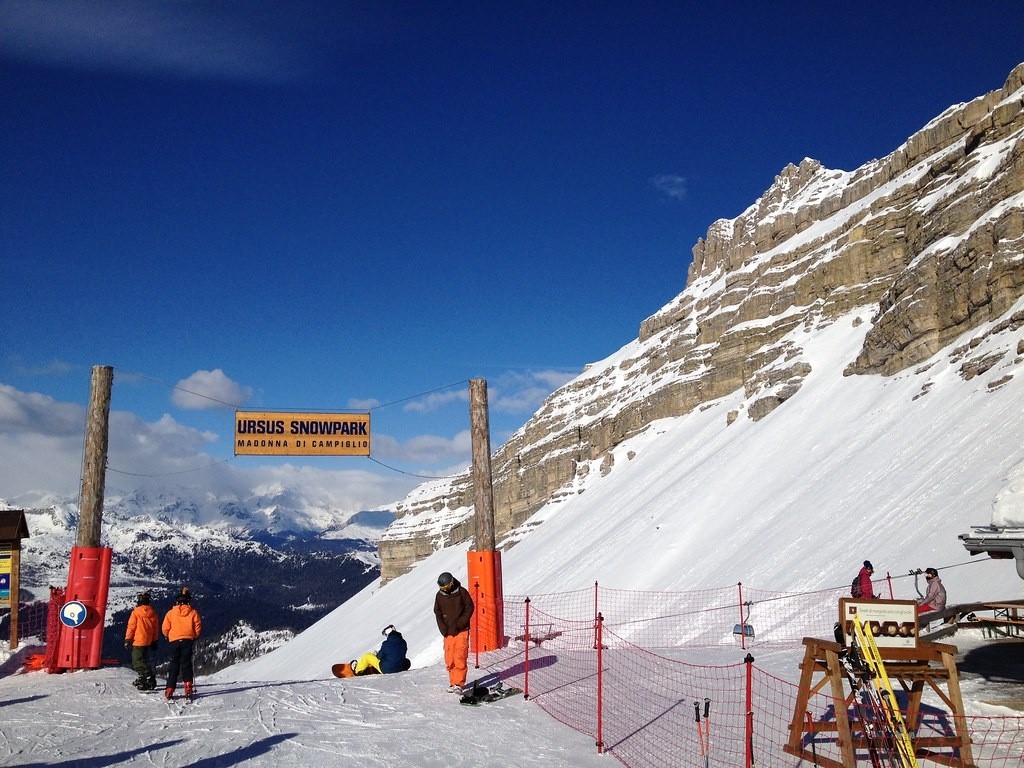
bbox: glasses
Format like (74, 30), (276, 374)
(440, 583), (451, 590)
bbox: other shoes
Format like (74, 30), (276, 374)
(134, 676), (157, 690)
(350, 660), (361, 676)
(372, 650), (377, 655)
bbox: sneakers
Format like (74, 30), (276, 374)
(446, 685), (463, 695)
(165, 688), (174, 700)
(184, 682), (193, 699)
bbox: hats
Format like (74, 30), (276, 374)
(176, 591), (191, 603)
(863, 560), (872, 568)
(138, 593), (151, 604)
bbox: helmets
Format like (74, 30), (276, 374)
(437, 572), (453, 586)
(925, 568), (938, 576)
(382, 624), (396, 636)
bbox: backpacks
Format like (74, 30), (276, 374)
(463, 680), (489, 701)
(851, 575), (861, 598)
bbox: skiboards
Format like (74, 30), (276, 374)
(831, 613), (918, 768)
(164, 694), (193, 704)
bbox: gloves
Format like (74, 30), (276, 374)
(150, 641), (159, 649)
(125, 642), (130, 651)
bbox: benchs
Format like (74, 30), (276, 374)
(972, 615), (1024, 640)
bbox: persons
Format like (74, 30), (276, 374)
(918, 567), (943, 613)
(350, 625), (407, 674)
(124, 590), (157, 691)
(860, 561), (876, 598)
(162, 588), (201, 699)
(433, 572), (474, 692)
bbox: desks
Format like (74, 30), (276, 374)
(983, 604), (1024, 620)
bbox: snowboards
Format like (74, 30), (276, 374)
(331, 658), (411, 679)
(132, 679), (158, 693)
